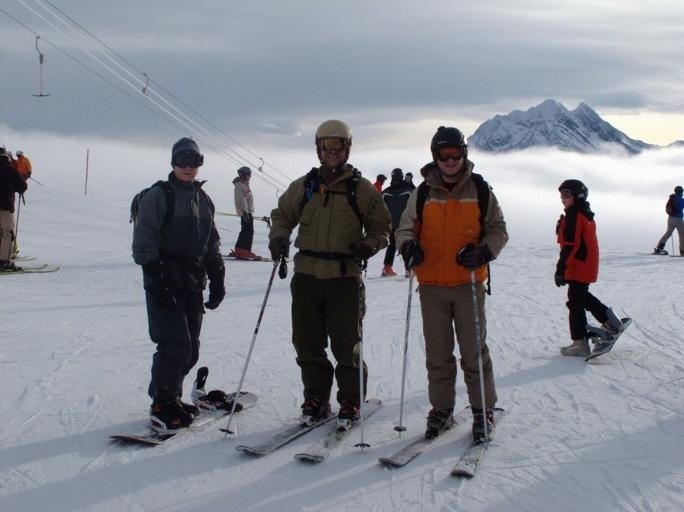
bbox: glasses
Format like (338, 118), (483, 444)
(435, 145), (464, 161)
(318, 138), (347, 152)
(560, 189), (579, 199)
(174, 153), (203, 168)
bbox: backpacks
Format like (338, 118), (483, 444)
(666, 194), (681, 214)
(129, 180), (210, 234)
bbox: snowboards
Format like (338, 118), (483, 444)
(579, 312), (633, 361)
(109, 392), (258, 445)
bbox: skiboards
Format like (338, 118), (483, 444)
(378, 405), (505, 480)
(13, 255), (39, 262)
(0, 264), (60, 275)
(634, 245), (682, 257)
(234, 398), (381, 463)
(219, 251), (274, 261)
(366, 273), (411, 283)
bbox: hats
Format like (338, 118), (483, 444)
(172, 137), (200, 162)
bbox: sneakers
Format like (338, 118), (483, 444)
(235, 247), (238, 253)
(383, 266), (397, 276)
(301, 399), (331, 418)
(406, 270), (415, 278)
(657, 247), (668, 254)
(0, 260), (21, 272)
(236, 248), (257, 260)
(471, 406), (496, 437)
(427, 408), (454, 432)
(338, 403), (361, 421)
(176, 393), (200, 417)
(151, 393), (193, 429)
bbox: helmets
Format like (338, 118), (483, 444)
(558, 179), (588, 201)
(316, 119), (353, 146)
(431, 126), (468, 152)
(16, 151), (23, 156)
(675, 186), (683, 193)
(238, 166), (250, 176)
(377, 168), (413, 183)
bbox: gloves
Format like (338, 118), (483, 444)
(555, 265), (566, 287)
(158, 280), (178, 310)
(244, 214), (251, 224)
(398, 239), (421, 266)
(455, 246), (490, 267)
(350, 242), (376, 264)
(268, 237), (290, 261)
(204, 280), (225, 309)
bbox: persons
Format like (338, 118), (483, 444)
(402, 172), (416, 189)
(5, 150), (16, 168)
(554, 179), (623, 357)
(13, 151), (32, 182)
(268, 119), (394, 434)
(231, 166), (256, 258)
(372, 174), (388, 195)
(132, 136), (226, 430)
(0, 143), (27, 272)
(655, 185), (684, 257)
(381, 167), (415, 276)
(395, 125), (509, 441)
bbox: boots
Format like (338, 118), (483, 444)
(561, 333), (590, 357)
(601, 307), (624, 333)
